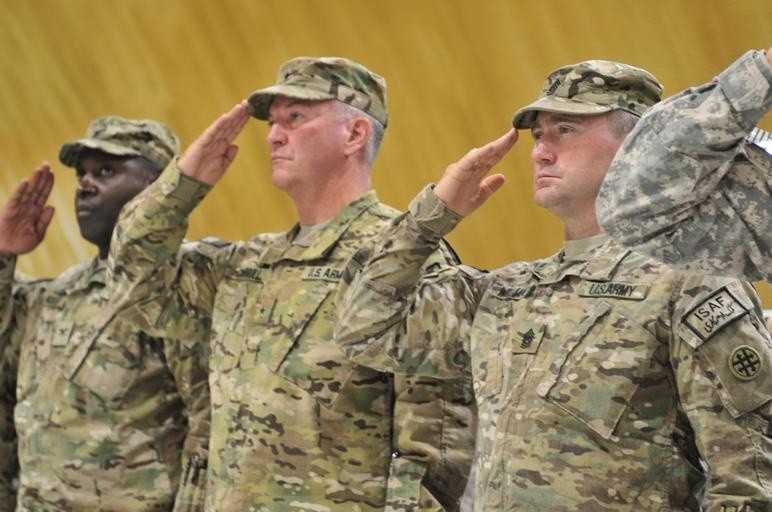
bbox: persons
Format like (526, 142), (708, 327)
(0, 115), (232, 511)
(331, 58), (772, 511)
(595, 44), (771, 279)
(104, 55), (477, 512)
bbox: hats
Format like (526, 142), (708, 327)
(56, 114), (181, 177)
(242, 55), (389, 133)
(510, 56), (665, 132)
